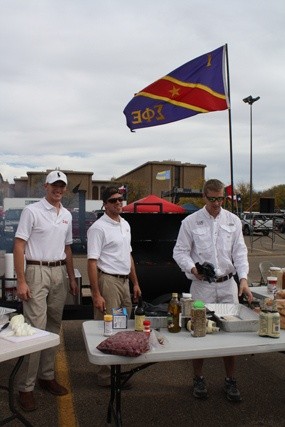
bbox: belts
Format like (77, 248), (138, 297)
(98, 268), (129, 279)
(212, 271), (233, 283)
(26, 259), (65, 267)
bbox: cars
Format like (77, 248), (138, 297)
(272, 209), (285, 233)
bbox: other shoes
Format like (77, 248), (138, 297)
(107, 377), (135, 390)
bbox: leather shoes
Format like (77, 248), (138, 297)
(38, 377), (67, 395)
(19, 391), (36, 412)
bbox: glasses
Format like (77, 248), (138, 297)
(206, 195), (224, 202)
(105, 197), (123, 203)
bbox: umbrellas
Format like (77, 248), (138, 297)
(123, 195), (198, 212)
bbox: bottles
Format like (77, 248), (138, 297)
(135, 294), (145, 331)
(103, 315), (113, 336)
(180, 293), (193, 317)
(167, 292), (182, 333)
(191, 302), (206, 337)
(142, 320), (150, 338)
(184, 319), (220, 333)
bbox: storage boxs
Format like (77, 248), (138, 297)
(204, 302), (260, 332)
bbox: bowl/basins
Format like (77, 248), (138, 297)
(203, 303), (260, 333)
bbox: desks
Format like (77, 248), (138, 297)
(0, 325), (60, 427)
(81, 315), (285, 427)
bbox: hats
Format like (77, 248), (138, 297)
(46, 170), (67, 185)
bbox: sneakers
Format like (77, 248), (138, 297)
(223, 377), (242, 401)
(193, 375), (208, 398)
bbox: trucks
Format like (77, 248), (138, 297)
(0, 197), (128, 253)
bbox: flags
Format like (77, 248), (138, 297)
(155, 169), (171, 181)
(225, 184), (237, 203)
(123, 45), (229, 132)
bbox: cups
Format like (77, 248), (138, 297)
(267, 277), (277, 292)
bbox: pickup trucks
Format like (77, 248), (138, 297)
(237, 211), (274, 235)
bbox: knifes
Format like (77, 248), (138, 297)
(0, 322), (10, 333)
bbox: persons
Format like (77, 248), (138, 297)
(13, 171), (80, 412)
(173, 179), (253, 403)
(86, 186), (142, 387)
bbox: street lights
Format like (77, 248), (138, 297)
(242, 95), (261, 212)
